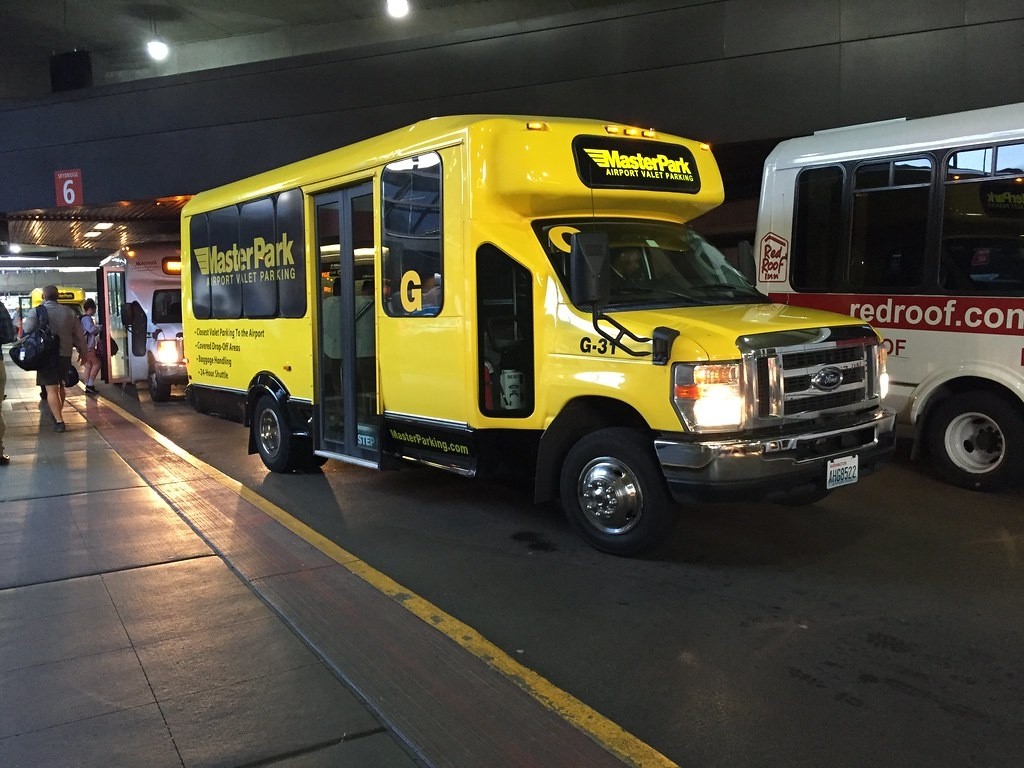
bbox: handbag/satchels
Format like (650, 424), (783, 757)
(9, 305), (60, 371)
(97, 331), (118, 356)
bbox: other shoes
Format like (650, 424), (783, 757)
(85, 391), (91, 393)
(55, 422), (65, 432)
(0, 455), (9, 464)
(86, 385), (99, 393)
(40, 392), (47, 399)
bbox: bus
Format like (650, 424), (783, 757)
(181, 114), (898, 557)
(96, 240), (189, 403)
(752, 102), (1024, 492)
(19, 287), (85, 320)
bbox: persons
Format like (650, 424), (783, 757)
(324, 279), (374, 430)
(22, 286), (88, 431)
(79, 300), (102, 394)
(0, 301), (19, 465)
